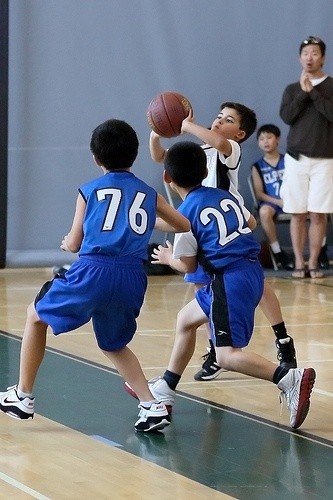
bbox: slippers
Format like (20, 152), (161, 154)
(292, 268), (306, 278)
(310, 268), (323, 277)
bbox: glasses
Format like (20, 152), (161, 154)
(303, 36), (322, 43)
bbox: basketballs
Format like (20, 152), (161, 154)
(146, 91), (194, 138)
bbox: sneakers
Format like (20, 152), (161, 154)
(1, 384), (34, 420)
(275, 368), (315, 429)
(273, 339), (295, 386)
(124, 379), (175, 411)
(194, 339), (231, 380)
(135, 404), (169, 433)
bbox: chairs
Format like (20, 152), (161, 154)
(162, 169), (183, 248)
(248, 173), (332, 270)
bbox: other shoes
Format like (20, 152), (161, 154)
(277, 248), (294, 270)
(319, 248), (328, 268)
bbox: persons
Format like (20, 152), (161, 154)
(1, 119), (193, 435)
(280, 36), (333, 281)
(250, 124), (327, 271)
(148, 102), (299, 382)
(123, 143), (316, 429)
